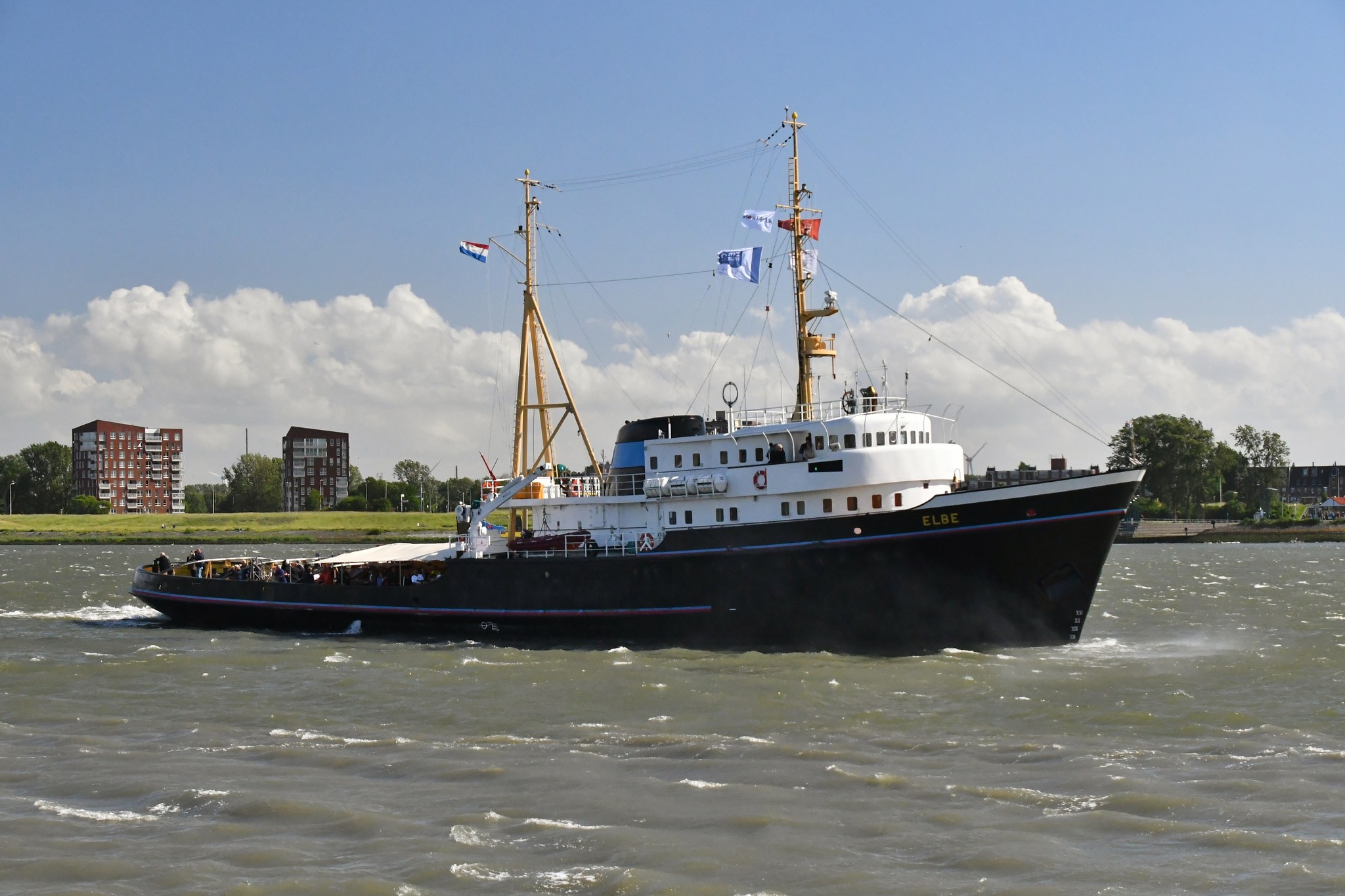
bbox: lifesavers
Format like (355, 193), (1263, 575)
(639, 533), (654, 551)
(571, 480), (582, 497)
(753, 471), (767, 489)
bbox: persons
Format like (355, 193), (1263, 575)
(1308, 514), (1312, 520)
(1291, 513), (1294, 520)
(766, 443), (786, 463)
(229, 558), (260, 581)
(1185, 527), (1188, 535)
(273, 552), (449, 586)
(1303, 514), (1306, 519)
(153, 552), (173, 575)
(1318, 509), (1345, 521)
(162, 523), (164, 528)
(172, 523), (176, 529)
(799, 436), (824, 461)
(187, 548), (204, 578)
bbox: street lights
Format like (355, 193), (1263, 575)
(364, 481), (368, 509)
(462, 492), (464, 503)
(213, 482), (216, 516)
(10, 482), (15, 516)
(287, 482), (291, 513)
(320, 479), (323, 511)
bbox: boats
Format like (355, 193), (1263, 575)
(120, 110), (1153, 654)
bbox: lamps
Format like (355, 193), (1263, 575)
(860, 386), (876, 397)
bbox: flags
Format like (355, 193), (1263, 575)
(716, 246), (763, 284)
(778, 218), (821, 241)
(741, 210), (775, 233)
(787, 249), (819, 275)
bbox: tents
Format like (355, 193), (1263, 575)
(307, 542), (470, 585)
